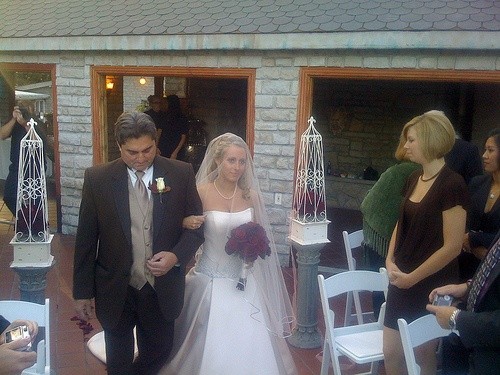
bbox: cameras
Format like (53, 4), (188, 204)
(430, 293), (455, 315)
(5, 324), (33, 352)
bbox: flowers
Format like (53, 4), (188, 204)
(70, 316), (92, 341)
(148, 177), (171, 203)
(225, 221), (272, 291)
(293, 192), (326, 215)
(14, 205), (50, 235)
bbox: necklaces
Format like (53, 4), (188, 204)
(421, 170), (439, 182)
(213, 177), (237, 200)
(489, 193), (497, 200)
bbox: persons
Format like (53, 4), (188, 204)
(381, 109), (469, 375)
(362, 163), (424, 321)
(0, 315), (39, 375)
(426, 230), (500, 374)
(183, 133), (300, 375)
(459, 128), (500, 282)
(0, 99), (47, 230)
(143, 94), (189, 158)
(72, 111), (205, 375)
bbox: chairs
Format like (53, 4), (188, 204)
(317, 268), (389, 375)
(397, 314), (459, 375)
(0, 297), (51, 375)
(343, 230), (374, 326)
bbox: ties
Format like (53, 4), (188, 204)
(134, 171), (149, 217)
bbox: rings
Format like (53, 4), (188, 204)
(193, 224), (196, 227)
(194, 220), (196, 223)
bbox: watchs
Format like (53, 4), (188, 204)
(449, 309), (460, 329)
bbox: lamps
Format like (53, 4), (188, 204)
(107, 82), (114, 95)
